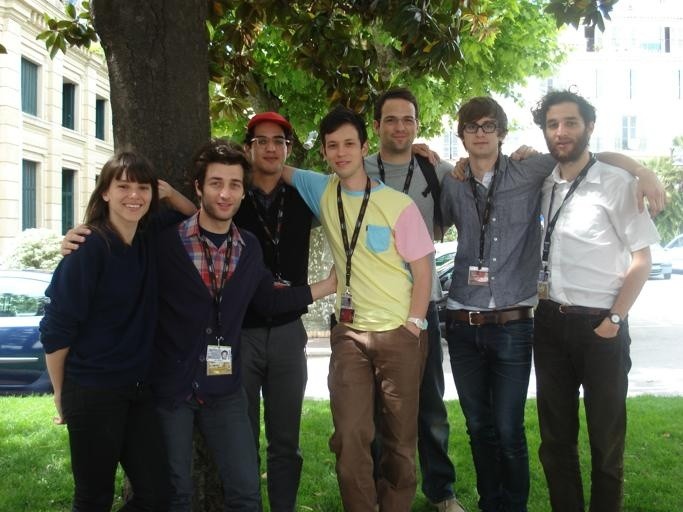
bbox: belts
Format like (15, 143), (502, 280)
(450, 307), (534, 326)
(542, 300), (610, 315)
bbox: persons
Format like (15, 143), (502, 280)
(282, 106), (437, 512)
(361, 86), (544, 512)
(61, 142), (340, 510)
(205, 111), (441, 511)
(37, 150), (199, 512)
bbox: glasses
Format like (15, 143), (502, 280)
(250, 137), (290, 147)
(463, 122), (496, 133)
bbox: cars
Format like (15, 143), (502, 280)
(434, 240), (457, 322)
(0, 269), (54, 397)
(646, 242), (671, 280)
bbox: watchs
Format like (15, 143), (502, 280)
(407, 317), (423, 328)
(607, 311), (626, 327)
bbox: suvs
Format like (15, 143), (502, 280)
(663, 233), (683, 277)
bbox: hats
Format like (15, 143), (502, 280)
(247, 112), (291, 128)
